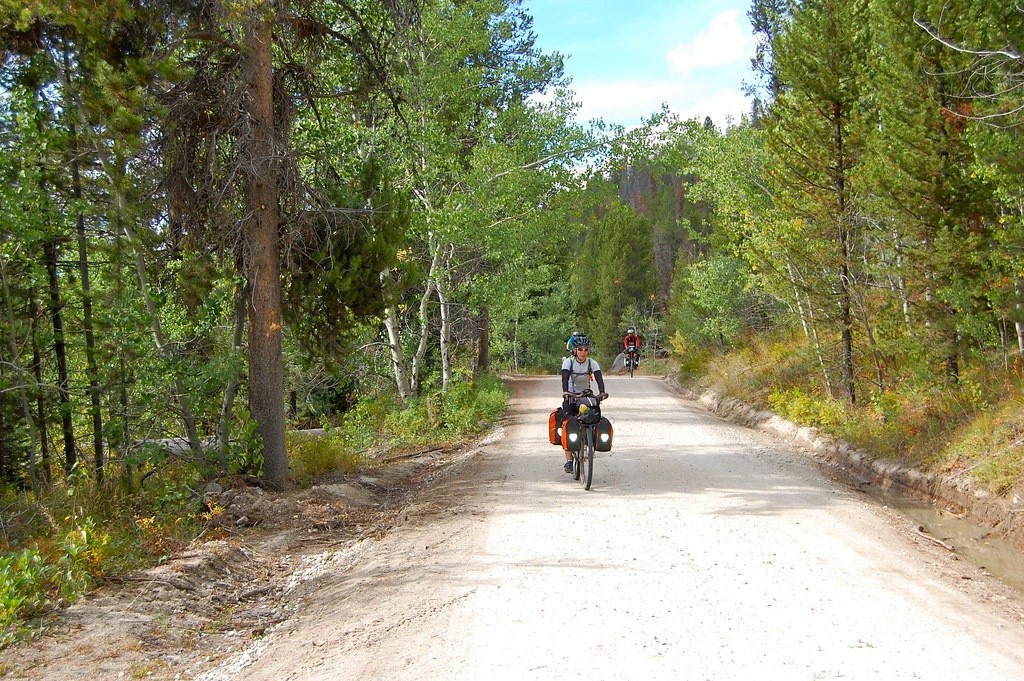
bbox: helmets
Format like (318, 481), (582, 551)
(627, 328), (635, 333)
(572, 333), (591, 346)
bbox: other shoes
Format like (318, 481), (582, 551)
(564, 461), (573, 473)
(626, 366), (630, 372)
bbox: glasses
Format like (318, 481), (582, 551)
(576, 348), (588, 351)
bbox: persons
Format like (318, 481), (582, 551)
(567, 332), (578, 359)
(562, 336), (605, 472)
(625, 329), (639, 372)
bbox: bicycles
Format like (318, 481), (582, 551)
(627, 346), (637, 377)
(562, 389), (609, 492)
